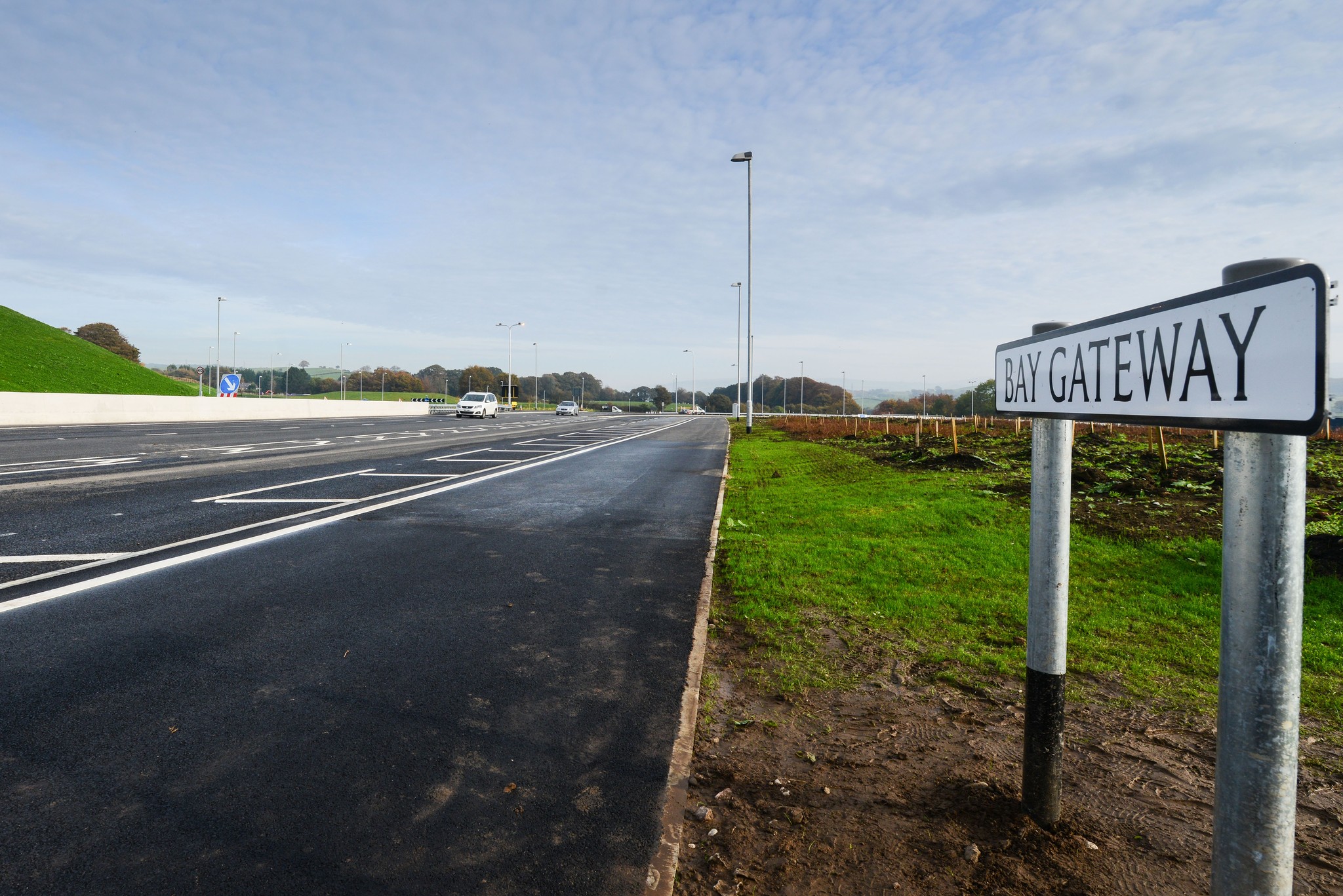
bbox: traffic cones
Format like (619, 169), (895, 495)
(518, 404), (523, 411)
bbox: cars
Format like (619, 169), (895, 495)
(680, 409), (692, 414)
(456, 392), (498, 419)
(556, 401), (579, 416)
(688, 406), (705, 415)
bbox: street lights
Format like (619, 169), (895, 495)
(922, 375), (926, 420)
(683, 350), (695, 415)
(629, 399), (631, 412)
(730, 282), (741, 422)
(580, 404), (583, 410)
(533, 343), (537, 412)
(496, 322), (525, 405)
(862, 380), (865, 415)
(762, 374), (766, 414)
(969, 381), (978, 418)
(671, 373), (677, 414)
(688, 408), (690, 415)
(730, 152), (754, 435)
(842, 371), (845, 416)
(784, 378), (787, 413)
(582, 377), (584, 406)
(195, 297), (489, 404)
(798, 361), (803, 415)
(500, 381), (503, 405)
(544, 390), (545, 408)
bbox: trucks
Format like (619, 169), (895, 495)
(600, 406), (623, 413)
